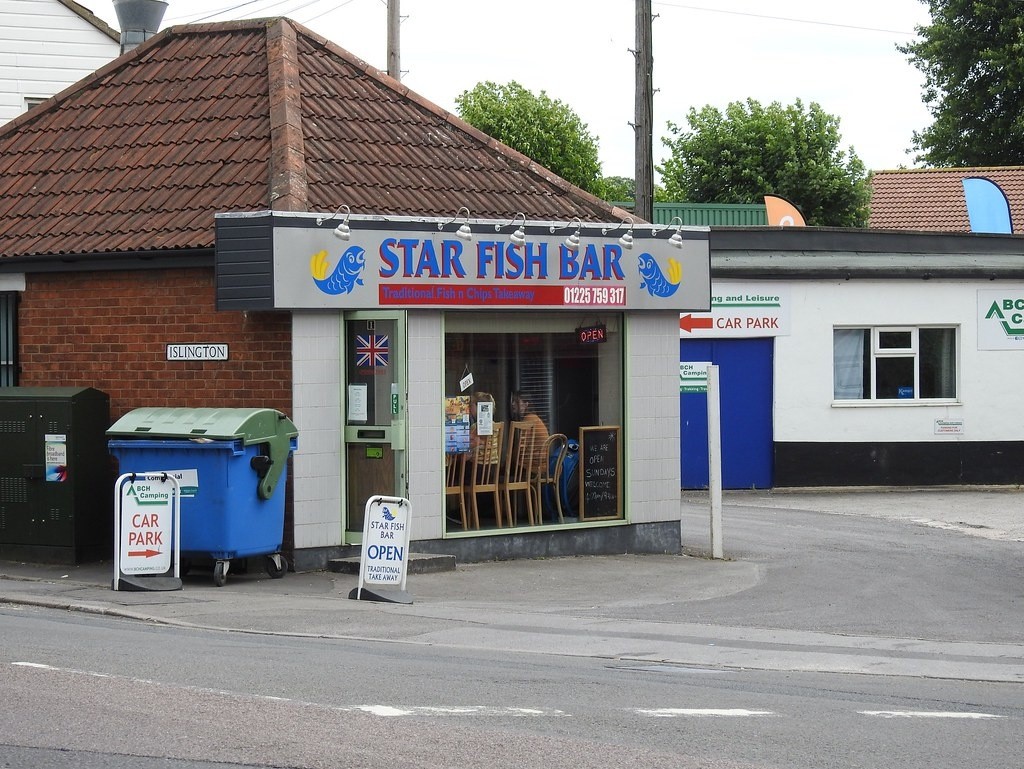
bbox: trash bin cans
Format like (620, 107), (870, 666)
(105, 406), (299, 585)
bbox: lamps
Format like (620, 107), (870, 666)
(651, 217), (684, 249)
(549, 217), (582, 252)
(437, 207), (472, 241)
(495, 212), (527, 246)
(601, 217), (634, 250)
(316, 205), (353, 241)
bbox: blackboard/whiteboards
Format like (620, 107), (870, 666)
(580, 426), (621, 519)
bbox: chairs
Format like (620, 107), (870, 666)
(446, 420), (569, 531)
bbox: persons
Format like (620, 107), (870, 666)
(446, 392), (499, 523)
(501, 390), (551, 520)
